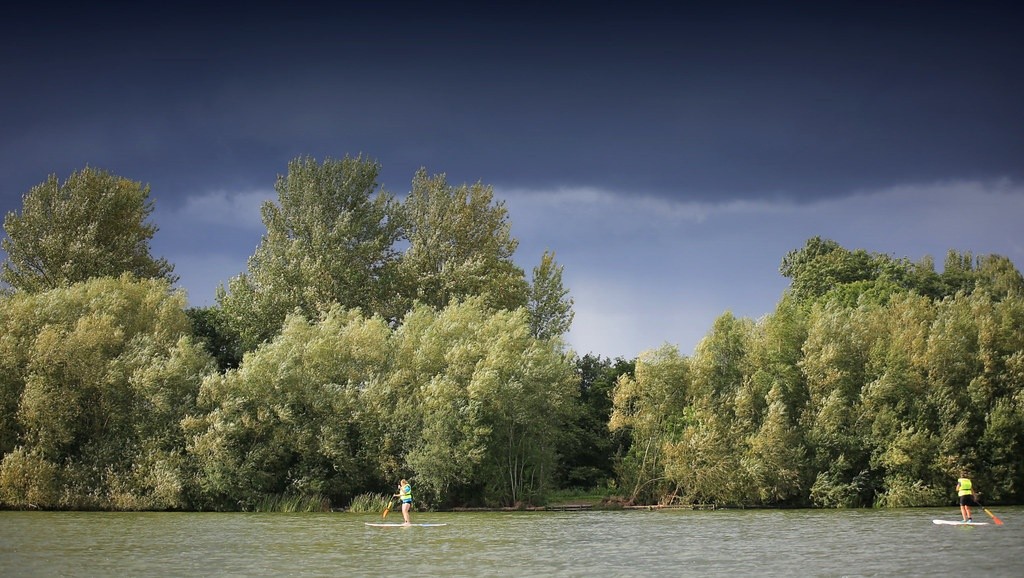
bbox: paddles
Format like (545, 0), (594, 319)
(382, 488), (399, 519)
(974, 500), (1004, 525)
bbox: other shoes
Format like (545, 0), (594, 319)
(962, 520), (967, 523)
(968, 518), (972, 523)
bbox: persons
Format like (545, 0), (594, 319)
(394, 479), (412, 525)
(955, 471), (978, 523)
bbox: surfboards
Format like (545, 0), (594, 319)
(364, 521), (448, 527)
(932, 519), (990, 526)
